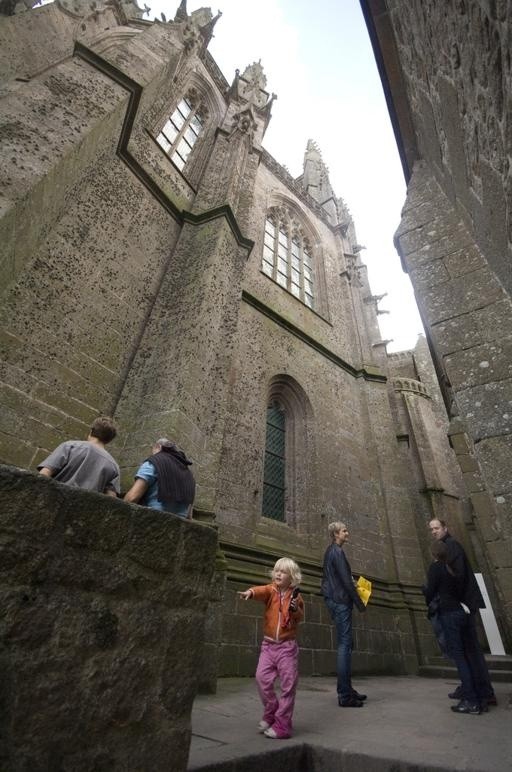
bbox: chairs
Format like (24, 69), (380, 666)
(339, 690), (367, 707)
(264, 727), (277, 738)
(258, 719), (269, 732)
(448, 687), (497, 714)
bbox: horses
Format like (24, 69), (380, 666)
(289, 588), (299, 612)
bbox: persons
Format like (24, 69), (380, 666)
(36, 416), (121, 498)
(427, 517), (497, 705)
(236, 557), (305, 740)
(123, 438), (196, 521)
(320, 521), (368, 708)
(424, 541), (489, 714)
